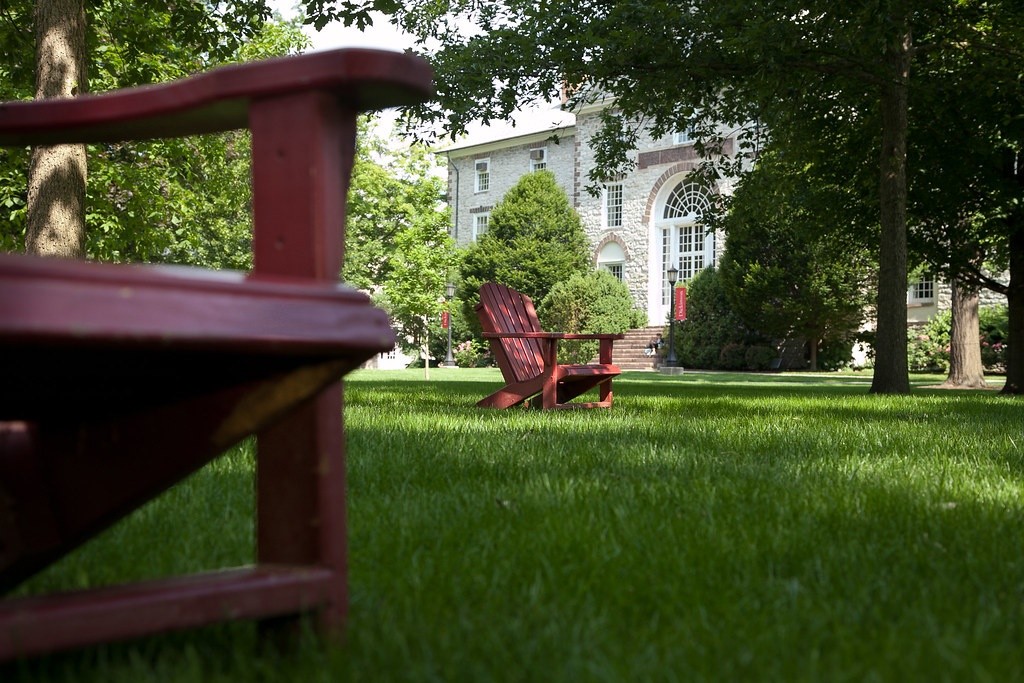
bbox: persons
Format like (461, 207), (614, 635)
(647, 333), (664, 355)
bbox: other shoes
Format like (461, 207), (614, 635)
(651, 351), (656, 355)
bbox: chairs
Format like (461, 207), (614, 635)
(0, 48), (434, 658)
(474, 282), (625, 412)
